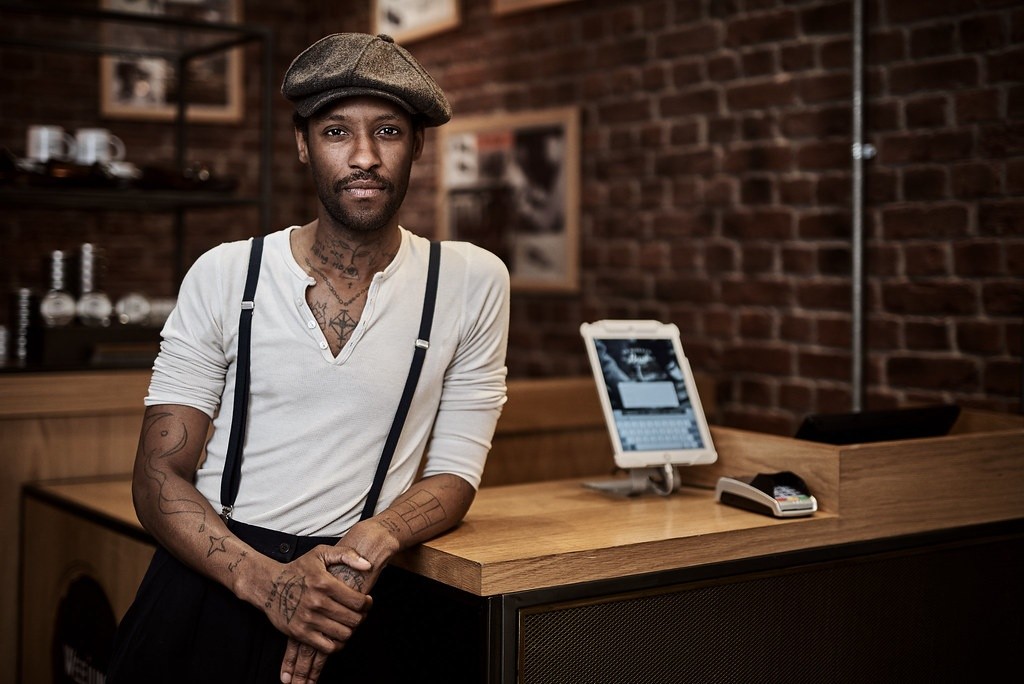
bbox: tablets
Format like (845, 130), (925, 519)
(579, 319), (719, 469)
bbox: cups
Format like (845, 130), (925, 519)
(73, 128), (129, 166)
(27, 125), (76, 162)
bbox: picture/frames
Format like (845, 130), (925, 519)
(372, 0), (566, 48)
(427, 104), (582, 296)
(100, 0), (247, 126)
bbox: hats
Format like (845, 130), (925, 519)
(279, 32), (452, 126)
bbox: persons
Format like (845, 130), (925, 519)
(118, 34), (511, 684)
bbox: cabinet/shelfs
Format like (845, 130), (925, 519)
(0, 0), (277, 374)
